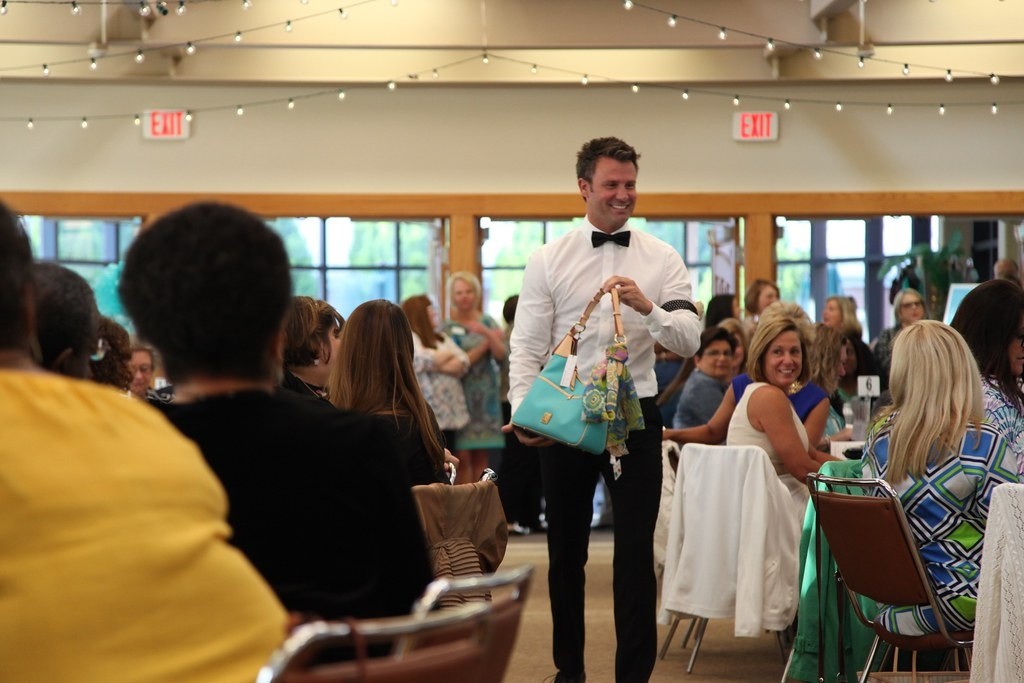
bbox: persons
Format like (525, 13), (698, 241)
(1, 185), (546, 683)
(499, 134), (700, 683)
(652, 257), (1024, 683)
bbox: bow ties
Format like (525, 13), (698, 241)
(592, 231), (631, 247)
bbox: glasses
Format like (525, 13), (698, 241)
(704, 350), (733, 359)
(89, 337), (111, 361)
(901, 302), (920, 308)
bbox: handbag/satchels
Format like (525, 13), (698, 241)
(511, 286), (645, 455)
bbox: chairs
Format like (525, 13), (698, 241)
(396, 564), (534, 660)
(660, 443), (804, 674)
(412, 468), (509, 609)
(256, 599), (489, 683)
(807, 473), (975, 683)
